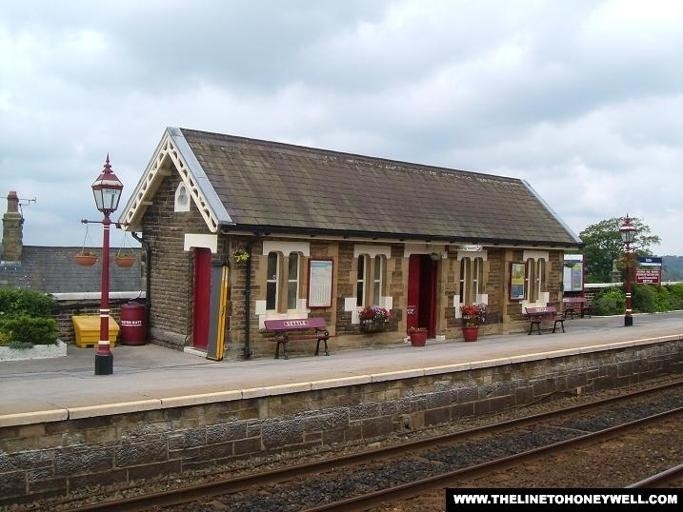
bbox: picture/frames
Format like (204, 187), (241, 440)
(306, 258), (334, 309)
(508, 261), (526, 302)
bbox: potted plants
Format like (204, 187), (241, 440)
(408, 326), (427, 346)
(114, 253), (136, 267)
(73, 249), (96, 266)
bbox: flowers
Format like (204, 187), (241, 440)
(359, 304), (391, 321)
(459, 304), (482, 327)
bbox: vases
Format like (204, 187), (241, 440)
(462, 328), (478, 342)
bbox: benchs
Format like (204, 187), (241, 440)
(262, 317), (332, 361)
(564, 297), (592, 319)
(525, 307), (566, 335)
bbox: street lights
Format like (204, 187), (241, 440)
(619, 212), (636, 326)
(80, 152), (131, 377)
